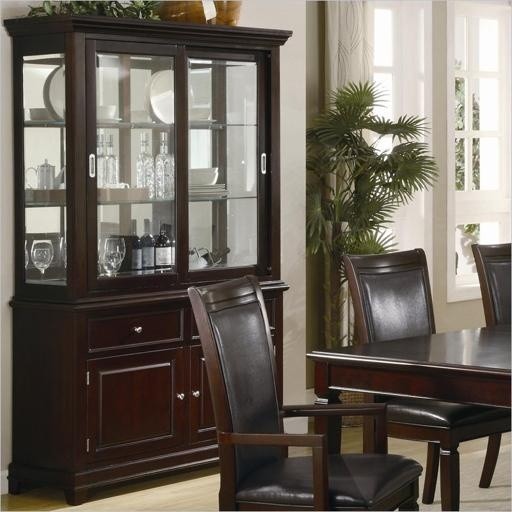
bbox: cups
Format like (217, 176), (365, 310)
(97, 104), (117, 118)
(29, 108), (50, 120)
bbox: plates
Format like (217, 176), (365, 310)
(188, 185), (228, 201)
(46, 67), (99, 120)
(97, 116), (122, 125)
(148, 70), (193, 125)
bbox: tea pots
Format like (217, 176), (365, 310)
(24, 160), (68, 191)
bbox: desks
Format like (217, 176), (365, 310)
(303, 319), (512, 511)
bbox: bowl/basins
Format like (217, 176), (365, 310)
(186, 166), (220, 184)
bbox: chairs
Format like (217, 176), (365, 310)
(469, 238), (511, 490)
(340, 246), (505, 502)
(183, 273), (425, 511)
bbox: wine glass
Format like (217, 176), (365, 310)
(30, 238), (55, 283)
(98, 235), (126, 277)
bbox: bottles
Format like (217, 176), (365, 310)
(95, 130), (175, 200)
(122, 217), (174, 270)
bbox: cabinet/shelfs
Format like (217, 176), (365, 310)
(2, 15), (289, 507)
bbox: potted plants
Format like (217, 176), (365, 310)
(307, 78), (440, 434)
